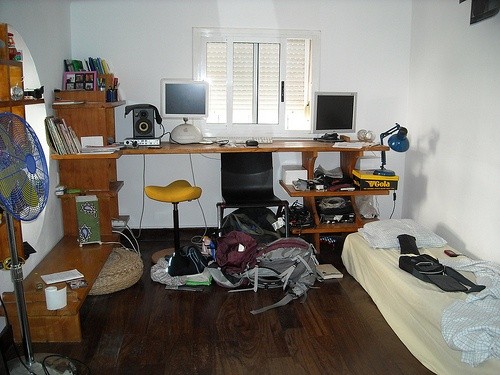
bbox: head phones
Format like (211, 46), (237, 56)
(357, 129), (376, 142)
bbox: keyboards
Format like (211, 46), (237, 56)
(217, 136), (273, 144)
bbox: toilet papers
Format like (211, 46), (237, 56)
(44, 286), (67, 310)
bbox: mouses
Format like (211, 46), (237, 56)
(246, 140), (259, 146)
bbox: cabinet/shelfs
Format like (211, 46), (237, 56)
(0, 89), (390, 342)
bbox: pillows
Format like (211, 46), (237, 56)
(358, 219), (448, 249)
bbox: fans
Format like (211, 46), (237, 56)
(0, 112), (78, 375)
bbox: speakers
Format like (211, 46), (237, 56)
(132, 108), (155, 139)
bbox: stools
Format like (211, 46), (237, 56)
(143, 180), (201, 263)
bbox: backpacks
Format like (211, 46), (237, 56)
(215, 209), (318, 314)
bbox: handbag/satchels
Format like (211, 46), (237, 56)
(168, 246), (208, 277)
(397, 234), (486, 294)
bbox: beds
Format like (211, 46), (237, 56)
(341, 233), (500, 375)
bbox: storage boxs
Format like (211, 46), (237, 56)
(352, 170), (400, 190)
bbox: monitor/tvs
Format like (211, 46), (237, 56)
(159, 77), (210, 143)
(313, 92), (357, 141)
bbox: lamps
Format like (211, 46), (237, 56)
(373, 123), (409, 176)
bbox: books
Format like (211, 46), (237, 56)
(80, 146), (119, 155)
(45, 116), (82, 155)
(64, 57), (111, 87)
(315, 264), (343, 279)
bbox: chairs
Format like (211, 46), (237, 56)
(216, 152), (290, 239)
